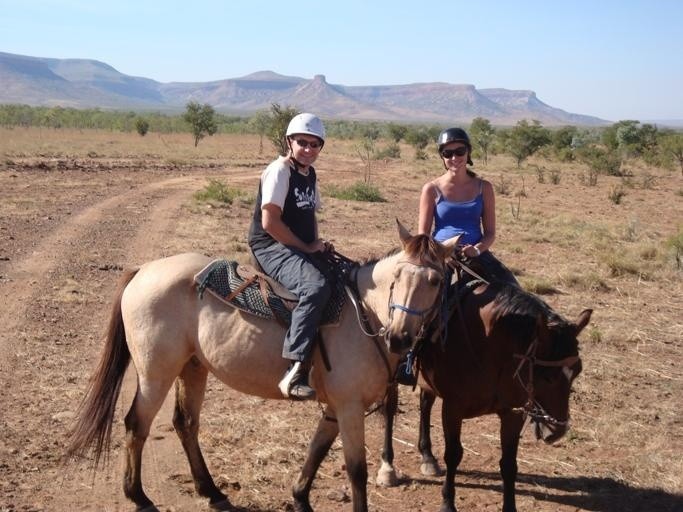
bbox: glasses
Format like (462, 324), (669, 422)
(440, 144), (468, 159)
(290, 136), (323, 149)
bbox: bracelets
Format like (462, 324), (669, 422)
(472, 245), (480, 257)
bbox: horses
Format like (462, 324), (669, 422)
(375, 244), (593, 512)
(61, 215), (466, 512)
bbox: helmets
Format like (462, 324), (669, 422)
(283, 111), (327, 143)
(435, 126), (474, 154)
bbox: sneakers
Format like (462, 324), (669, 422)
(279, 367), (318, 400)
(394, 358), (419, 386)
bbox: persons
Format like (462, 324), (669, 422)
(246, 112), (338, 401)
(395, 127), (497, 387)
